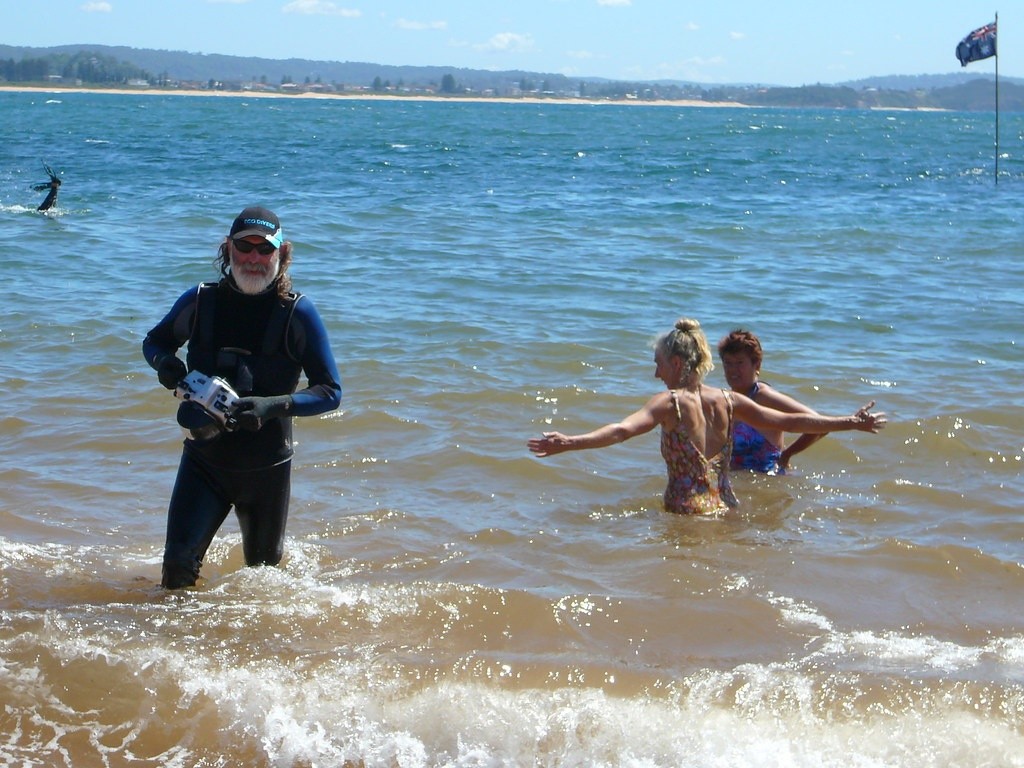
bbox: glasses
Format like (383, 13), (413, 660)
(228, 237), (276, 256)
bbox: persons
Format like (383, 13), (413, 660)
(142, 207), (342, 589)
(526, 317), (888, 515)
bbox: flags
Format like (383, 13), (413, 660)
(955, 22), (997, 67)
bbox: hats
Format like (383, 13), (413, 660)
(229, 207), (284, 250)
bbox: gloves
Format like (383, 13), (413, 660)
(230, 394), (293, 432)
(154, 351), (187, 390)
